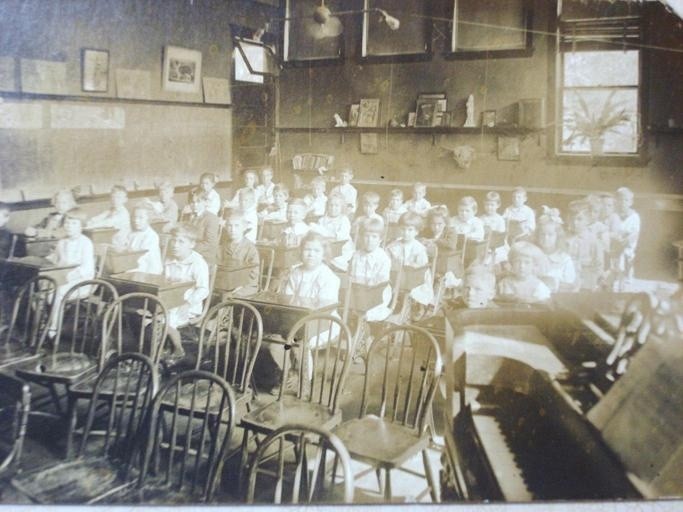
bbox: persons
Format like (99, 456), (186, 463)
(18, 163), (640, 367)
(264, 232), (338, 395)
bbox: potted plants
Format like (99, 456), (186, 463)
(561, 87), (637, 156)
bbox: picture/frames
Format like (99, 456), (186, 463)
(498, 135), (518, 161)
(160, 44), (200, 93)
(412, 93), (446, 117)
(443, 0), (534, 59)
(359, 98), (380, 128)
(276, 1), (347, 70)
(357, 0), (436, 63)
(414, 98), (448, 128)
(79, 47), (109, 94)
(347, 103), (359, 128)
(358, 132), (378, 155)
(481, 110), (497, 129)
(516, 97), (545, 128)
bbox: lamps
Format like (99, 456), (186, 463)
(251, 0), (400, 47)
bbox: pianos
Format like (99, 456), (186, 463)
(423, 305), (683, 502)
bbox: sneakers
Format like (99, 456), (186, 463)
(158, 348), (185, 370)
(269, 373), (313, 400)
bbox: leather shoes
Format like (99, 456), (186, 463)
(41, 329), (60, 351)
